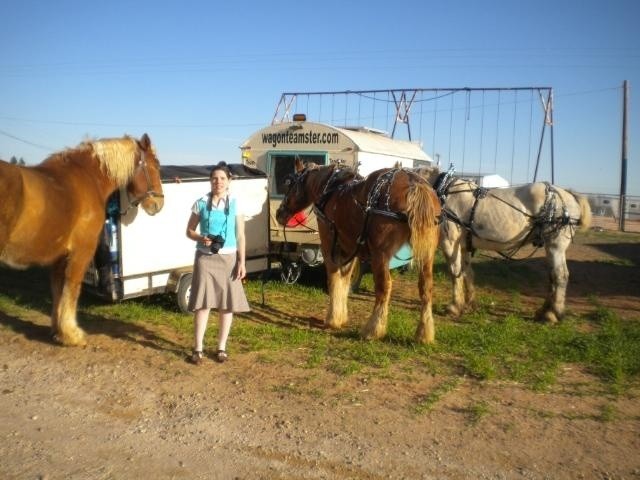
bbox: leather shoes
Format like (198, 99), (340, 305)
(214, 349), (227, 362)
(189, 350), (204, 364)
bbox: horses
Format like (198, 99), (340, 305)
(275, 154), (441, 343)
(394, 161), (592, 323)
(0, 133), (164, 348)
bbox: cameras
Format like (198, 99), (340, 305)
(207, 233), (225, 255)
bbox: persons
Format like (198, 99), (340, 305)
(186, 160), (251, 365)
